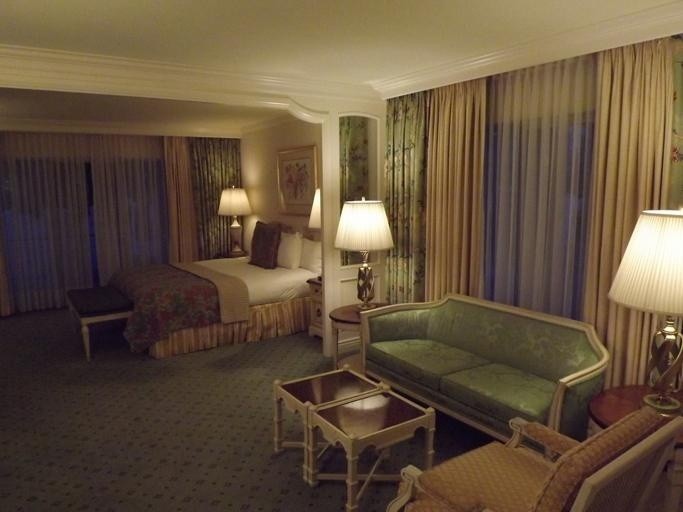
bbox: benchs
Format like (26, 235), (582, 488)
(65, 285), (133, 363)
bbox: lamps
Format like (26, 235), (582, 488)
(217, 186), (252, 256)
(334, 197), (395, 310)
(309, 188), (322, 281)
(607, 206), (683, 421)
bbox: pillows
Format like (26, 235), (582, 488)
(248, 220), (303, 270)
(533, 406), (663, 512)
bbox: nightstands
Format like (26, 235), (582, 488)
(306, 278), (323, 338)
(218, 251), (247, 258)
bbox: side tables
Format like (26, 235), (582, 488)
(328, 303), (392, 369)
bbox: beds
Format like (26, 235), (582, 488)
(103, 215), (323, 359)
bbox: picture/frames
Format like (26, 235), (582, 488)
(275, 145), (318, 216)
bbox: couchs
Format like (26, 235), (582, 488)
(359, 293), (611, 459)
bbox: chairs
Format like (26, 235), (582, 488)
(386, 406), (683, 512)
